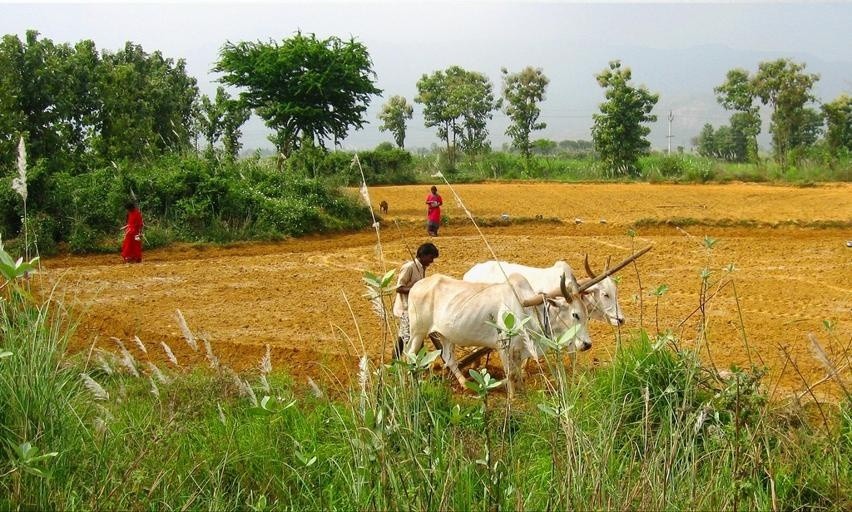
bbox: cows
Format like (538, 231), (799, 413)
(395, 271), (592, 401)
(462, 253), (626, 379)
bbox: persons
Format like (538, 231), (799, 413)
(425, 185), (443, 237)
(389, 242), (439, 375)
(120, 202), (144, 264)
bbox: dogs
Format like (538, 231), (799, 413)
(379, 201), (388, 212)
